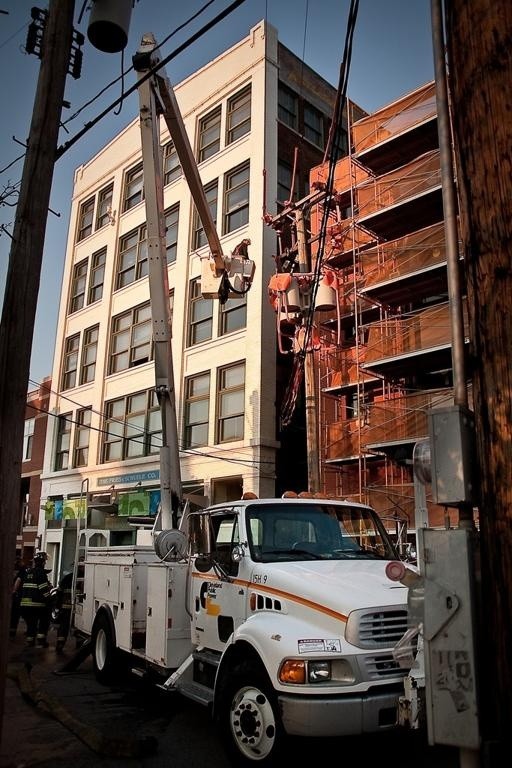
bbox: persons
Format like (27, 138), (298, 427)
(232, 236), (252, 261)
(10, 558), (33, 639)
(54, 555), (86, 658)
(18, 551), (54, 650)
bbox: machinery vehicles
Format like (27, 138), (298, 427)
(72, 32), (429, 767)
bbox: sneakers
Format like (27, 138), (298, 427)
(23, 641), (64, 656)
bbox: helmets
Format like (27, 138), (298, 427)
(33, 552), (49, 561)
